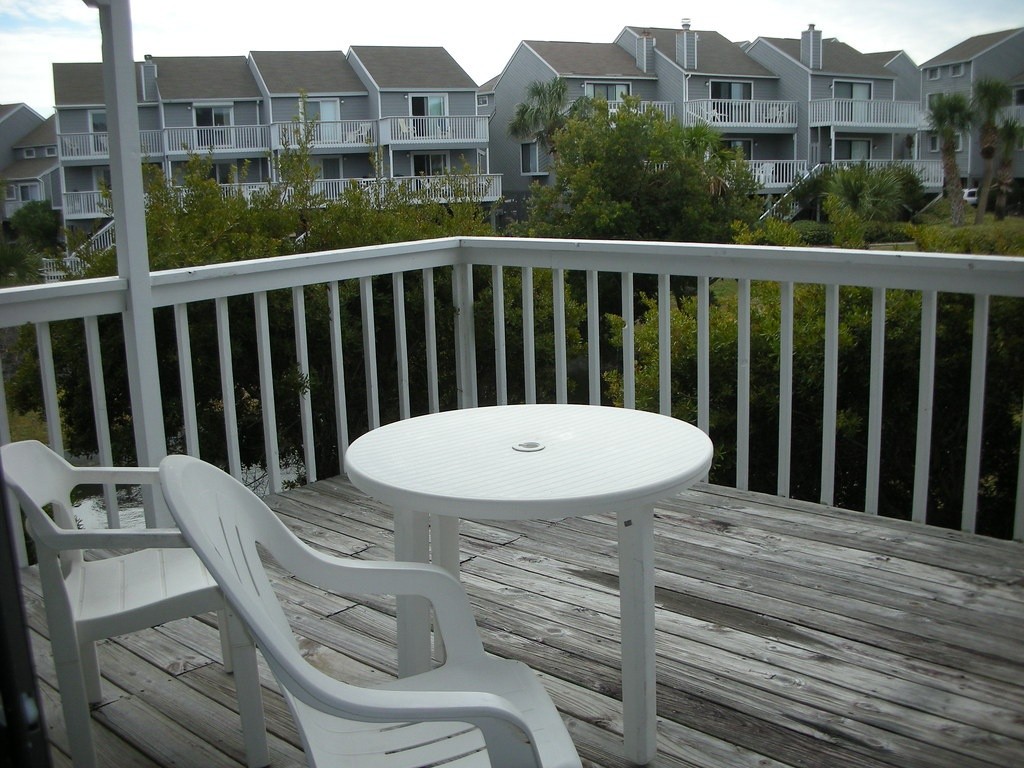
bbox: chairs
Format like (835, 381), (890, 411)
(60, 137), (80, 156)
(398, 119), (417, 140)
(438, 126), (450, 139)
(353, 123), (372, 143)
(0, 439), (271, 768)
(763, 105), (789, 123)
(159, 454), (583, 768)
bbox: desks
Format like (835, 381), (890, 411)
(345, 404), (716, 766)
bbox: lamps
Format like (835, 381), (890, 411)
(828, 144), (832, 148)
(754, 141), (758, 146)
(406, 152), (410, 157)
(404, 94), (408, 99)
(705, 82), (709, 86)
(829, 85), (833, 89)
(343, 156), (347, 160)
(873, 145), (878, 149)
